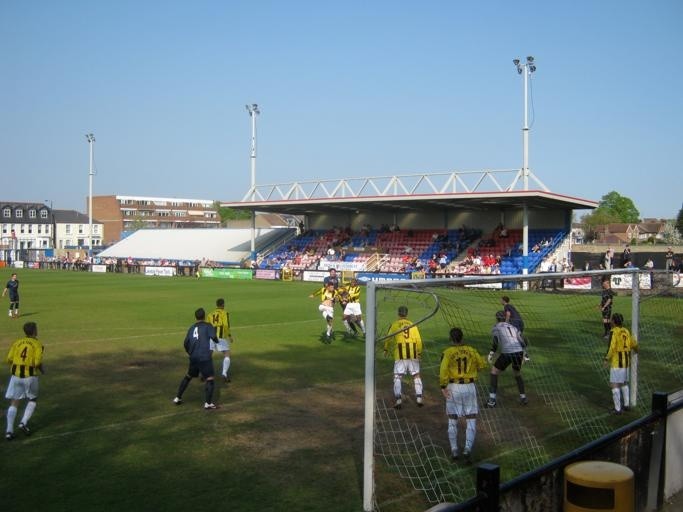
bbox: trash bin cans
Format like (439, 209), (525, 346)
(563, 461), (634, 512)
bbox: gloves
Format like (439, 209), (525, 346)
(487, 350), (496, 366)
(522, 351), (530, 364)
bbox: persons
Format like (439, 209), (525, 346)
(3, 322), (46, 441)
(239, 221), (515, 274)
(2, 273), (20, 320)
(173, 300), (234, 408)
(148, 258), (214, 277)
(602, 244), (676, 271)
(308, 269), (366, 341)
(531, 237), (549, 253)
(599, 280), (639, 415)
(382, 296), (530, 457)
(547, 257), (592, 272)
(37, 257), (132, 274)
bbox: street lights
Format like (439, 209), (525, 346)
(43, 199), (53, 249)
(244, 103), (260, 270)
(83, 131), (96, 258)
(512, 52), (538, 293)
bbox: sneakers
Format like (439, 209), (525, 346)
(18, 422), (31, 435)
(172, 396), (182, 405)
(463, 447), (474, 463)
(607, 405), (632, 416)
(203, 402), (216, 410)
(519, 394), (529, 407)
(603, 333), (611, 340)
(4, 431), (13, 439)
(221, 371), (230, 381)
(8, 313), (19, 318)
(325, 329), (367, 339)
(486, 397), (497, 408)
(450, 448), (460, 462)
(393, 398), (402, 410)
(416, 397), (424, 408)
